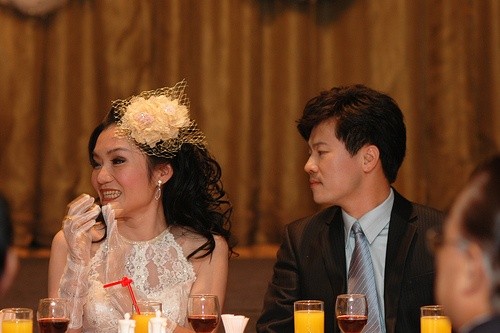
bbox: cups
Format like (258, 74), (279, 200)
(293, 299), (325, 333)
(187, 294), (221, 333)
(420, 306), (452, 333)
(132, 303), (162, 333)
(336, 294), (368, 333)
(37, 298), (70, 333)
(0, 307), (33, 333)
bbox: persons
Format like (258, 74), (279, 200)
(256, 85), (446, 333)
(47, 79), (240, 333)
(434, 152), (499, 333)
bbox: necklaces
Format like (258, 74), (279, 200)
(119, 226), (172, 244)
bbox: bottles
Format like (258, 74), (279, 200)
(148, 310), (167, 333)
(117, 312), (136, 333)
